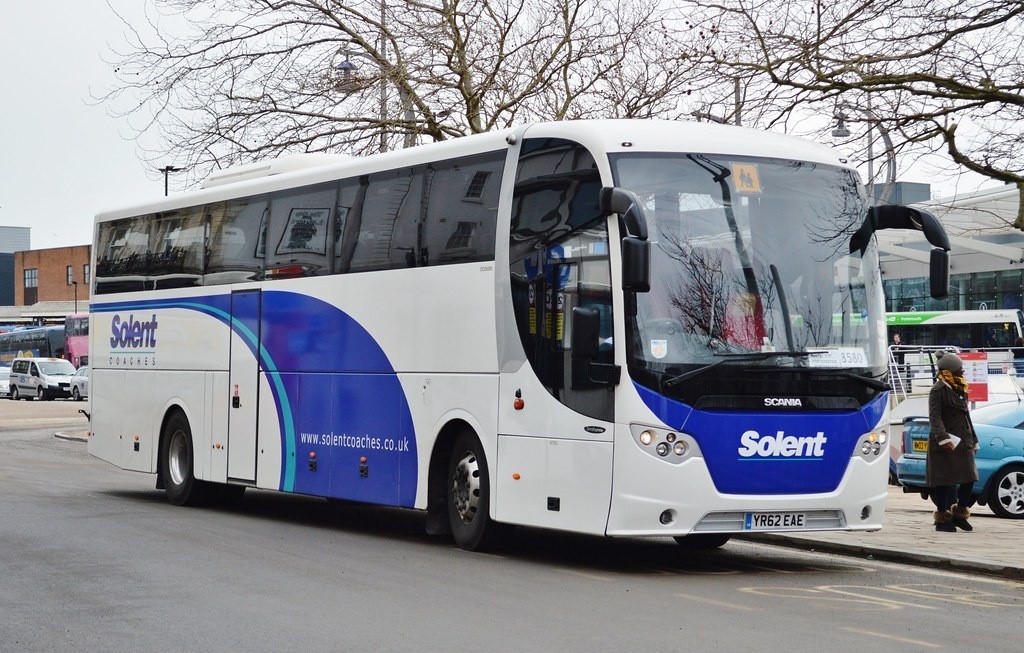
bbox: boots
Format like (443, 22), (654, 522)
(951, 505), (972, 530)
(934, 510), (957, 532)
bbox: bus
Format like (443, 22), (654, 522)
(0, 324), (67, 368)
(63, 312), (89, 371)
(786, 309), (1024, 389)
(82, 118), (954, 553)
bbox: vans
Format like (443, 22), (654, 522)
(8, 357), (78, 401)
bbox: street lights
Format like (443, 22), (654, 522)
(71, 280), (78, 314)
(832, 62), (875, 209)
(157, 165), (185, 196)
(336, 0), (387, 154)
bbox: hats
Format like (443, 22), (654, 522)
(935, 350), (963, 373)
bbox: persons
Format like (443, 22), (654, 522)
(721, 254), (774, 353)
(926, 352), (981, 531)
(891, 333), (907, 364)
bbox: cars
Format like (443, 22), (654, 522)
(888, 374), (1024, 520)
(0, 367), (14, 399)
(68, 365), (89, 400)
